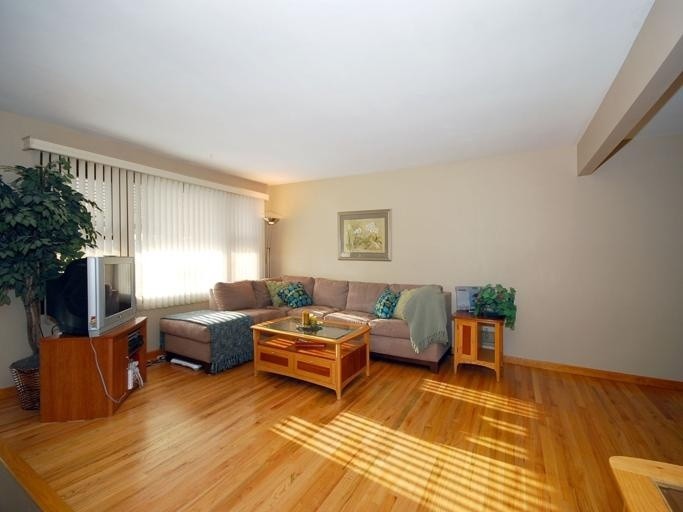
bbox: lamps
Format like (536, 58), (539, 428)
(263, 218), (279, 278)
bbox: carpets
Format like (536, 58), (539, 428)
(0, 461), (44, 512)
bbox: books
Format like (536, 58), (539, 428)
(295, 337), (327, 348)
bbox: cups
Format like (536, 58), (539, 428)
(301, 311), (318, 325)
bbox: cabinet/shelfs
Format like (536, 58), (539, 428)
(38, 316), (147, 422)
(451, 311), (506, 382)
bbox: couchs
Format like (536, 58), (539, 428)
(160, 289), (452, 374)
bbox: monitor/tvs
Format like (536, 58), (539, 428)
(41, 257), (136, 337)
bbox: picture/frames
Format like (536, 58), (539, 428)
(336, 209), (392, 261)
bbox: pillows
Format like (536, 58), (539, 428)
(208, 274), (442, 319)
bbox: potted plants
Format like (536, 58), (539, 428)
(472, 283), (517, 330)
(0, 155), (103, 410)
